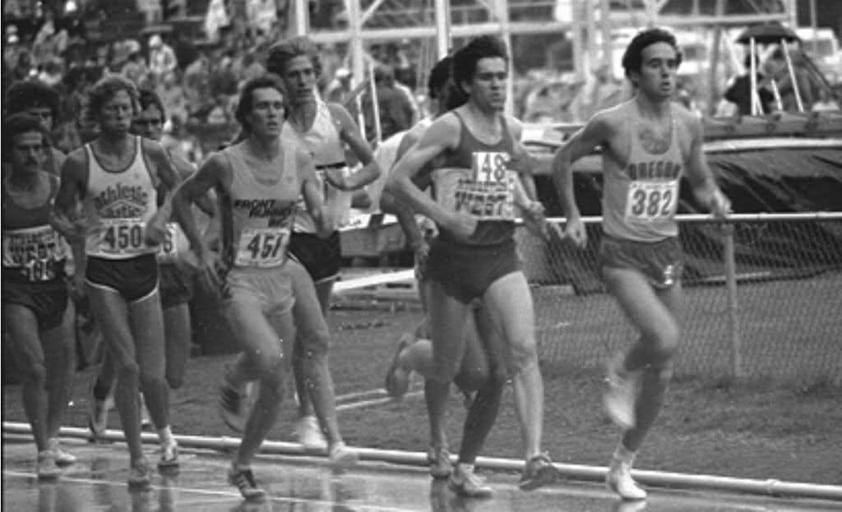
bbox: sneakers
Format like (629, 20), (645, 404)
(296, 415), (329, 453)
(603, 354), (640, 429)
(449, 467), (492, 496)
(518, 455), (557, 491)
(38, 450), (62, 478)
(157, 439), (178, 469)
(138, 391), (152, 426)
(86, 376), (109, 433)
(605, 470), (647, 499)
(227, 463), (265, 498)
(428, 440), (451, 479)
(48, 438), (75, 464)
(129, 456), (149, 486)
(329, 442), (355, 474)
(219, 362), (247, 433)
(385, 336), (411, 396)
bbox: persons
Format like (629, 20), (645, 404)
(330, 39), (558, 503)
(719, 36), (824, 117)
(3, 0), (381, 503)
(552, 28), (733, 504)
